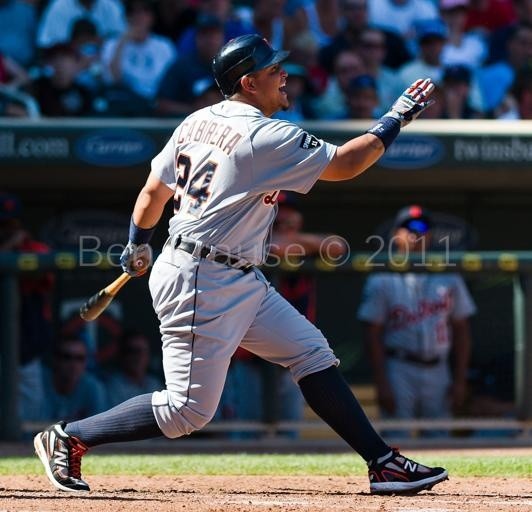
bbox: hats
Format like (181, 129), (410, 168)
(394, 203), (434, 230)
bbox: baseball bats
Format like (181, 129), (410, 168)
(79, 261), (142, 322)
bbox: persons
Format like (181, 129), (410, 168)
(1, 0), (532, 442)
(31, 31), (451, 496)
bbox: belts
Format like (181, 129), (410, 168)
(165, 235), (254, 276)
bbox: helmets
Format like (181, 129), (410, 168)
(209, 33), (291, 101)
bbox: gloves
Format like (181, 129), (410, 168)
(388, 78), (437, 130)
(118, 241), (153, 281)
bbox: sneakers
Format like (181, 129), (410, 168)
(365, 447), (450, 495)
(32, 418), (89, 494)
